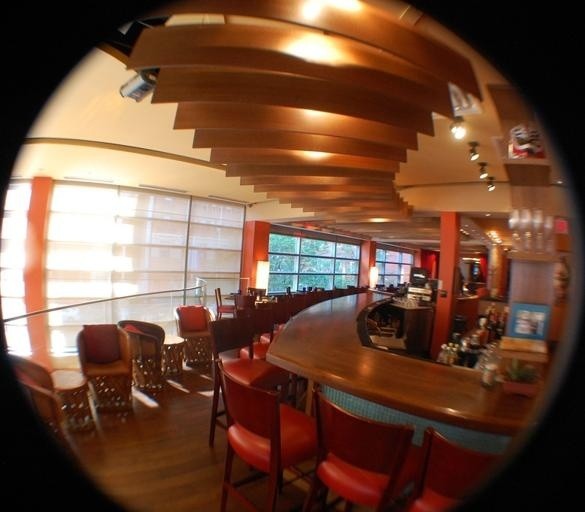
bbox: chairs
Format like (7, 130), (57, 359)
(216, 358), (319, 511)
(205, 319), (299, 446)
(312, 388), (426, 510)
(237, 323), (290, 361)
(406, 426), (507, 511)
(238, 282), (408, 323)
(9, 284), (237, 443)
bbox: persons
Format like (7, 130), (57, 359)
(303, 287), (317, 294)
(545, 257), (573, 353)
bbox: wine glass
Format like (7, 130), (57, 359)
(461, 223), (498, 251)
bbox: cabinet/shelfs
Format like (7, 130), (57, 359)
(494, 156), (565, 364)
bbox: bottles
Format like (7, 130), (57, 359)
(482, 349), (498, 388)
(436, 303), (510, 363)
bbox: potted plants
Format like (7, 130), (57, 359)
(502, 363), (541, 400)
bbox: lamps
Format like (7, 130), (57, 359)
(447, 115), (497, 195)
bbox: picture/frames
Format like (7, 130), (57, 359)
(508, 299), (552, 342)
